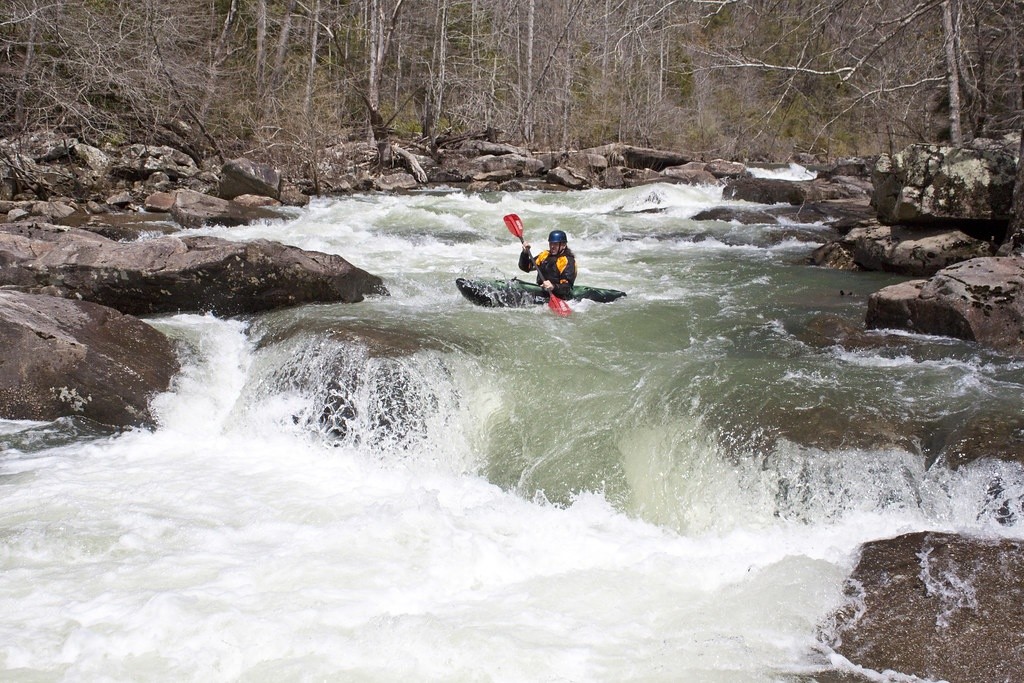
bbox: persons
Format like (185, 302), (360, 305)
(518, 229), (577, 298)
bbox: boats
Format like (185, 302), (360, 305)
(456, 278), (626, 309)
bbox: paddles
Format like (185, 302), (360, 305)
(504, 213), (573, 319)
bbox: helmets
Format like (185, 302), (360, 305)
(548, 230), (568, 243)
